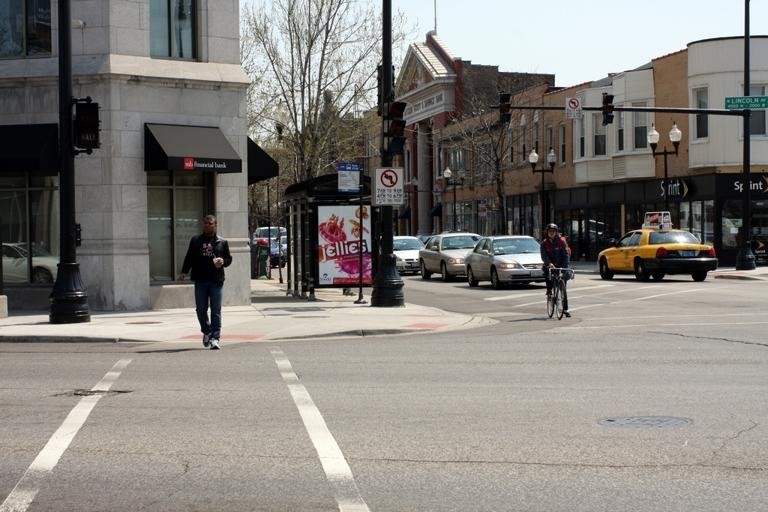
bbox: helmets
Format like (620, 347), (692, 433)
(546, 222), (558, 232)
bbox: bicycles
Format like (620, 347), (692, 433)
(545, 267), (574, 321)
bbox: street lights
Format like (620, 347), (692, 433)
(275, 98), (322, 181)
(443, 167), (465, 231)
(647, 120), (682, 211)
(529, 146), (557, 239)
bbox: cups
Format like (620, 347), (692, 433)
(212, 257), (222, 268)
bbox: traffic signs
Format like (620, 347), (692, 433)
(724, 96), (768, 110)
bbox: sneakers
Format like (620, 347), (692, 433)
(345, 290), (357, 296)
(208, 337), (221, 350)
(342, 289), (347, 295)
(562, 310), (571, 317)
(545, 290), (552, 295)
(202, 331), (212, 348)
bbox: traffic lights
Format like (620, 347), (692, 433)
(387, 102), (405, 154)
(605, 95), (614, 123)
(501, 93), (512, 122)
(78, 102), (99, 148)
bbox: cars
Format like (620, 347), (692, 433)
(446, 232), (481, 247)
(598, 210), (718, 282)
(464, 234), (546, 288)
(418, 232), (477, 280)
(393, 236), (427, 274)
(419, 234), (432, 243)
(253, 227), (289, 268)
(1, 242), (62, 285)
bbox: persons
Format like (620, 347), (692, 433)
(177, 215), (232, 349)
(540, 223), (571, 317)
